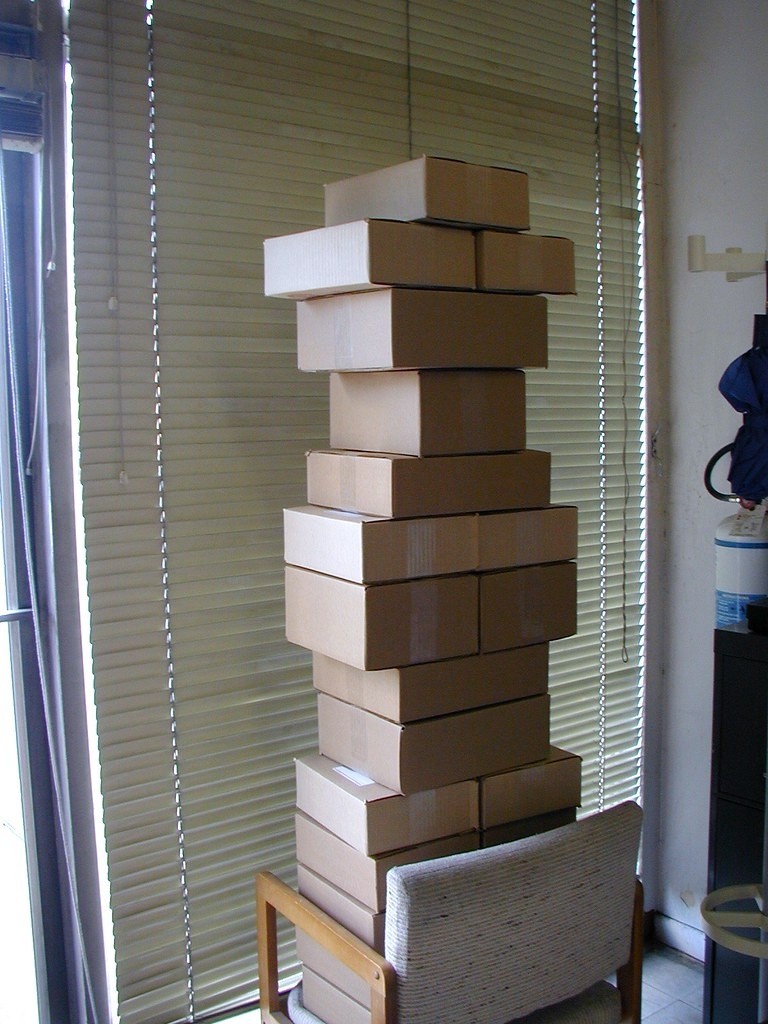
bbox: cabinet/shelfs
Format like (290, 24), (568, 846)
(700, 627), (767, 1024)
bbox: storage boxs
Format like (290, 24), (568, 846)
(261, 153), (582, 1024)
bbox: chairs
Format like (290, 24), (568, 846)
(255, 798), (645, 1024)
